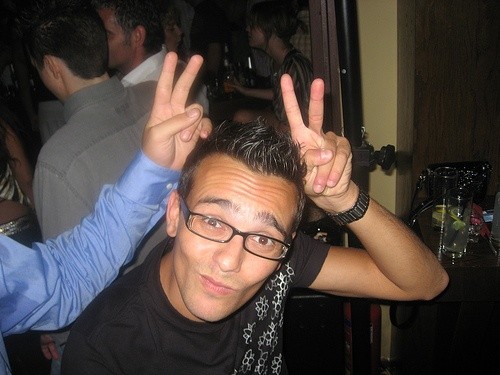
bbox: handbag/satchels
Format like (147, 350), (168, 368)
(427, 160), (490, 208)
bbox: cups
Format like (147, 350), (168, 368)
(490, 193), (500, 255)
(431, 163), (459, 229)
(441, 195), (474, 258)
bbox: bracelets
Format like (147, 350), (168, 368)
(323, 188), (370, 226)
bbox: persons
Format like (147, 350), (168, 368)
(60, 75), (449, 375)
(0, 52), (213, 375)
(0, 0), (311, 375)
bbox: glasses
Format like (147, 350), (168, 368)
(179, 195), (292, 261)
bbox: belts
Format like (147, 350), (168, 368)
(0, 213), (35, 236)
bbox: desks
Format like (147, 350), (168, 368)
(413, 199), (500, 372)
